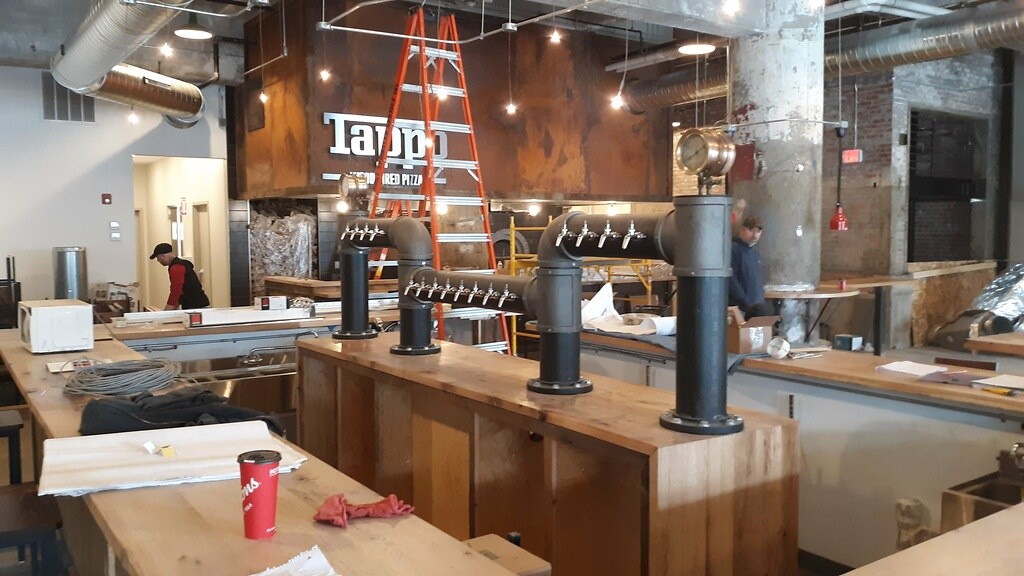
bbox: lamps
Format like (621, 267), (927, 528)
(829, 126), (849, 231)
(172, 0), (213, 40)
(678, 36), (716, 55)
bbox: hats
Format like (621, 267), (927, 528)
(743, 216), (764, 229)
(150, 243), (172, 259)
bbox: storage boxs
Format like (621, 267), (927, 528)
(833, 334), (864, 352)
(459, 533), (552, 576)
(727, 306), (780, 354)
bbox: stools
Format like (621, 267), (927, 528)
(0, 480), (63, 576)
(0, 409), (26, 562)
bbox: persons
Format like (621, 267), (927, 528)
(728, 215), (765, 316)
(149, 243), (210, 311)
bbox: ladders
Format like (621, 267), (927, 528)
(370, 7), (514, 355)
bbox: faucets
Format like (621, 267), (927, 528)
(597, 220), (623, 248)
(497, 284), (520, 307)
(554, 221), (577, 247)
(340, 223), (354, 241)
(440, 279), (458, 300)
(575, 220), (598, 247)
(359, 223), (373, 241)
(415, 277), (431, 297)
(622, 220), (647, 249)
(453, 279), (471, 302)
(369, 223), (386, 241)
(427, 277), (444, 299)
(1012, 442), (1020, 458)
(481, 282), (500, 305)
(349, 223), (364, 241)
(467, 281), (486, 303)
(403, 275), (420, 295)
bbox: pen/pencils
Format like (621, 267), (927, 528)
(792, 353), (822, 360)
(944, 370), (967, 374)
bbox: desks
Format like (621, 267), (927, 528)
(0, 261), (1024, 576)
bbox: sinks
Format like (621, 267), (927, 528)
(945, 471), (1024, 507)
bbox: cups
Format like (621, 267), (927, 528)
(840, 281), (846, 290)
(238, 450), (281, 540)
